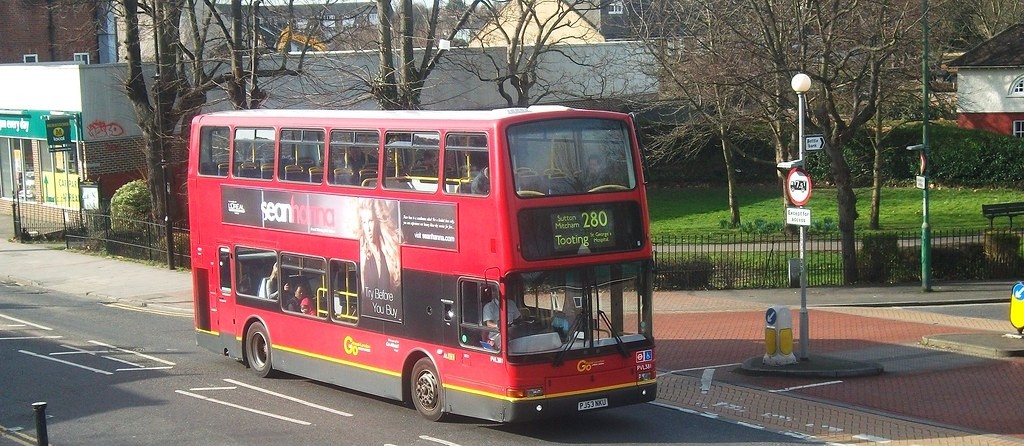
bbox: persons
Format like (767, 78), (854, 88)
(287, 284), (316, 317)
(352, 199), (401, 318)
(266, 262), (292, 300)
(584, 154), (602, 189)
(483, 286), (521, 341)
(347, 146), (377, 176)
(415, 149), (435, 177)
(471, 163), (489, 194)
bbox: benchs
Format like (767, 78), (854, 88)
(982, 202), (1024, 228)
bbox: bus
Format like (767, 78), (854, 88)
(187, 105), (657, 423)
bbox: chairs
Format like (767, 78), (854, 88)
(307, 167), (323, 182)
(217, 162), (227, 175)
(333, 168), (353, 185)
(359, 169), (378, 186)
(294, 157), (315, 173)
(284, 165), (296, 180)
(334, 271), (356, 304)
(514, 166), (583, 195)
(261, 163), (274, 178)
(237, 162), (258, 177)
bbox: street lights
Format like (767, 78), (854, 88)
(906, 144), (933, 292)
(777, 74), (812, 359)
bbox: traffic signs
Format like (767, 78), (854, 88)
(804, 135), (826, 151)
